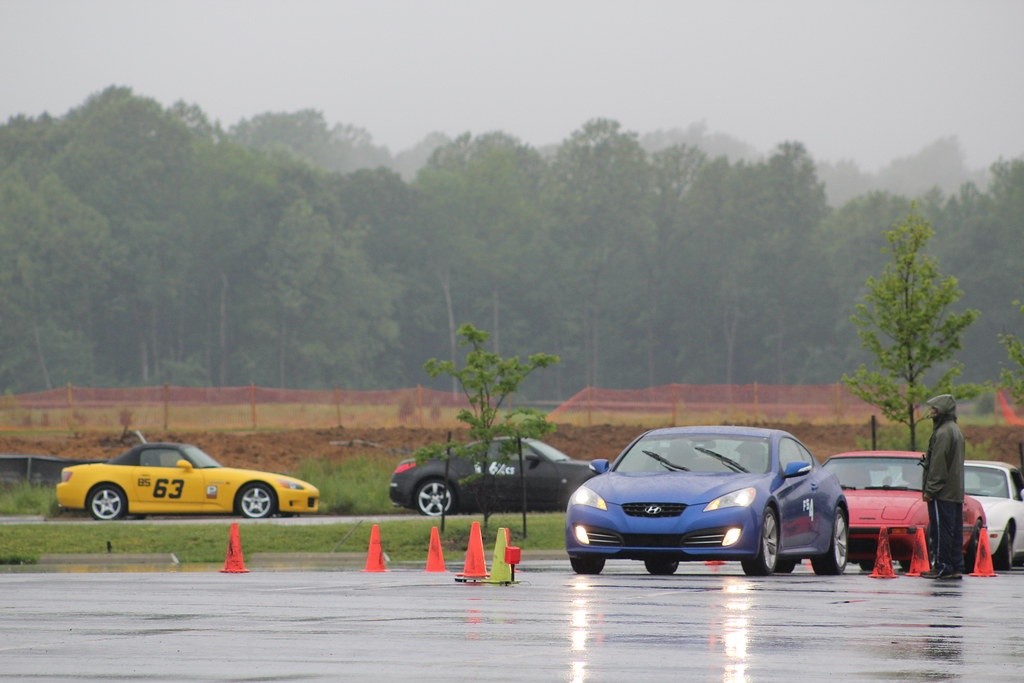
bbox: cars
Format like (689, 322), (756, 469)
(816, 450), (988, 574)
(962, 459), (1024, 570)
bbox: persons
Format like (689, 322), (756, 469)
(920, 396), (965, 580)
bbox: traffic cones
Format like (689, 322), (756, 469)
(705, 561), (728, 567)
(481, 527), (520, 583)
(967, 527), (1001, 578)
(359, 523), (390, 573)
(903, 524), (933, 576)
(426, 527), (447, 573)
(504, 527), (522, 571)
(453, 521), (492, 578)
(868, 524), (899, 578)
(219, 521), (252, 573)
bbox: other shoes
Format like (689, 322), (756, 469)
(941, 571), (963, 579)
(921, 568), (952, 579)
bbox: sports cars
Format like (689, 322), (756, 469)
(55, 442), (321, 521)
(388, 435), (603, 517)
(563, 425), (852, 576)
